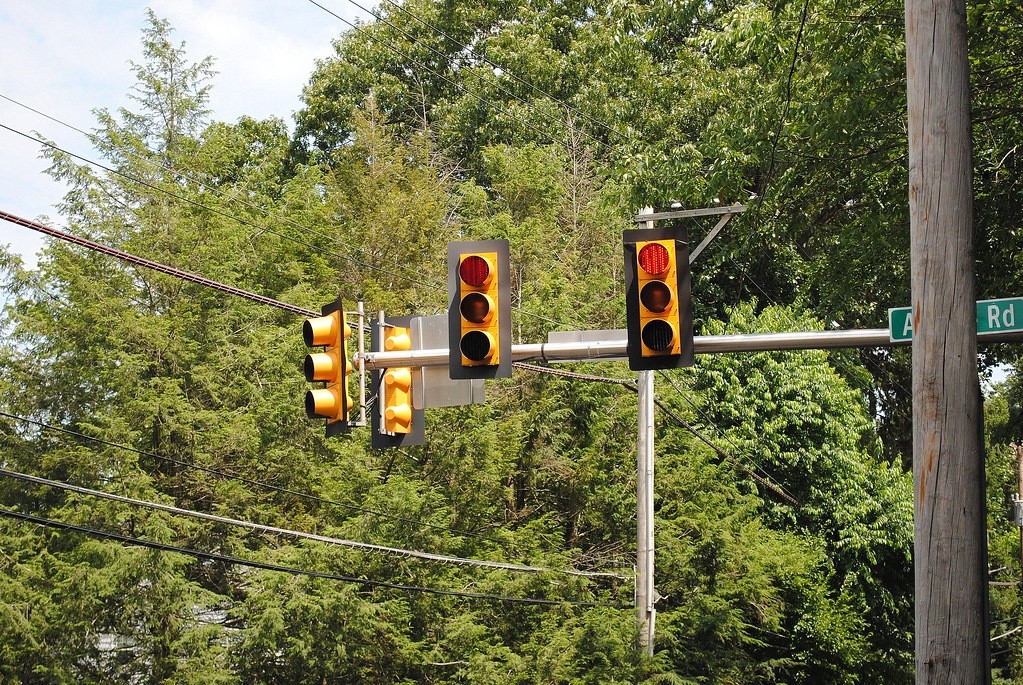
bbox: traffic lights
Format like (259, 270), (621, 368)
(303, 297), (353, 440)
(622, 225), (696, 372)
(446, 238), (513, 380)
